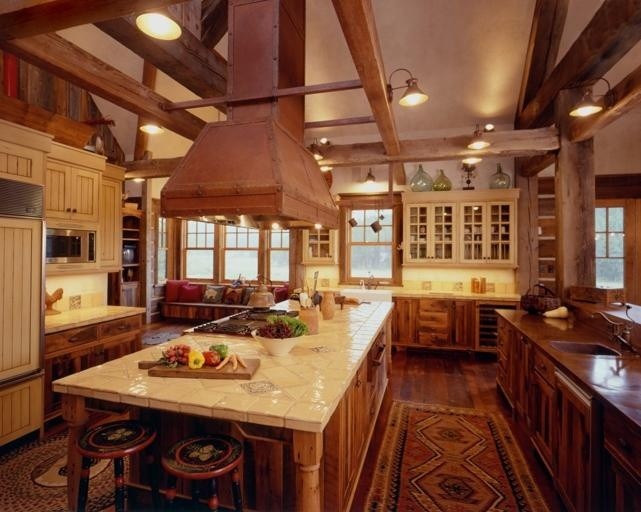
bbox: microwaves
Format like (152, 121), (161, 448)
(45, 227), (96, 264)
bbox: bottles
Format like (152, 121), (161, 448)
(411, 164), (433, 192)
(482, 277), (486, 294)
(312, 290), (322, 304)
(288, 294), (312, 310)
(541, 264), (556, 271)
(490, 163), (511, 188)
(473, 278), (480, 292)
(321, 290), (337, 319)
(435, 169), (452, 191)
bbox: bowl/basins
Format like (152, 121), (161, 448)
(250, 330), (302, 356)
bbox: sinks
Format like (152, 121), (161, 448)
(548, 340), (623, 359)
(340, 288), (393, 302)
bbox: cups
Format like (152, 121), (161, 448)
(348, 218), (358, 228)
(371, 221), (383, 232)
(301, 306), (320, 335)
(409, 225), (507, 242)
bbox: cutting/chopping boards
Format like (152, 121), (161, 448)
(139, 357), (261, 380)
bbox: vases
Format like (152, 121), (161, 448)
(430, 168), (454, 192)
(488, 161), (513, 190)
(407, 163), (434, 193)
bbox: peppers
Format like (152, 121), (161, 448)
(188, 350), (205, 369)
(209, 345), (228, 360)
(203, 351), (221, 366)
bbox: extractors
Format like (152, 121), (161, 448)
(161, 1), (341, 238)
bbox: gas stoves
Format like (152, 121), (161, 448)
(194, 309), (299, 338)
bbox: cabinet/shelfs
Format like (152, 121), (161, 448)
(494, 305), (598, 511)
(95, 163), (127, 275)
(390, 292), (517, 366)
(42, 303), (150, 428)
(400, 188), (523, 272)
(0, 117), (56, 453)
(600, 400), (641, 510)
(297, 225), (342, 266)
(41, 138), (108, 222)
(106, 207), (156, 308)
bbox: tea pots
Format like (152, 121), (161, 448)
(248, 275), (278, 308)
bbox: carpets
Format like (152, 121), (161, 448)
(1, 417), (132, 512)
(357, 394), (553, 512)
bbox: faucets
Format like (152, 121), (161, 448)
(367, 275), (375, 289)
(588, 311), (624, 344)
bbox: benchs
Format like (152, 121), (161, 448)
(157, 283), (285, 323)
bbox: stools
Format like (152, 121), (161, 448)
(71, 414), (165, 511)
(157, 430), (248, 510)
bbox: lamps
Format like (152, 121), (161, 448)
(135, 117), (166, 136)
(382, 63), (431, 110)
(566, 75), (619, 117)
(361, 167), (379, 189)
(305, 137), (325, 161)
(466, 122), (491, 149)
(135, 8), (185, 42)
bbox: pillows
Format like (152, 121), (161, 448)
(241, 286), (260, 305)
(220, 286), (246, 306)
(199, 284), (226, 305)
(175, 284), (202, 305)
(164, 278), (190, 302)
